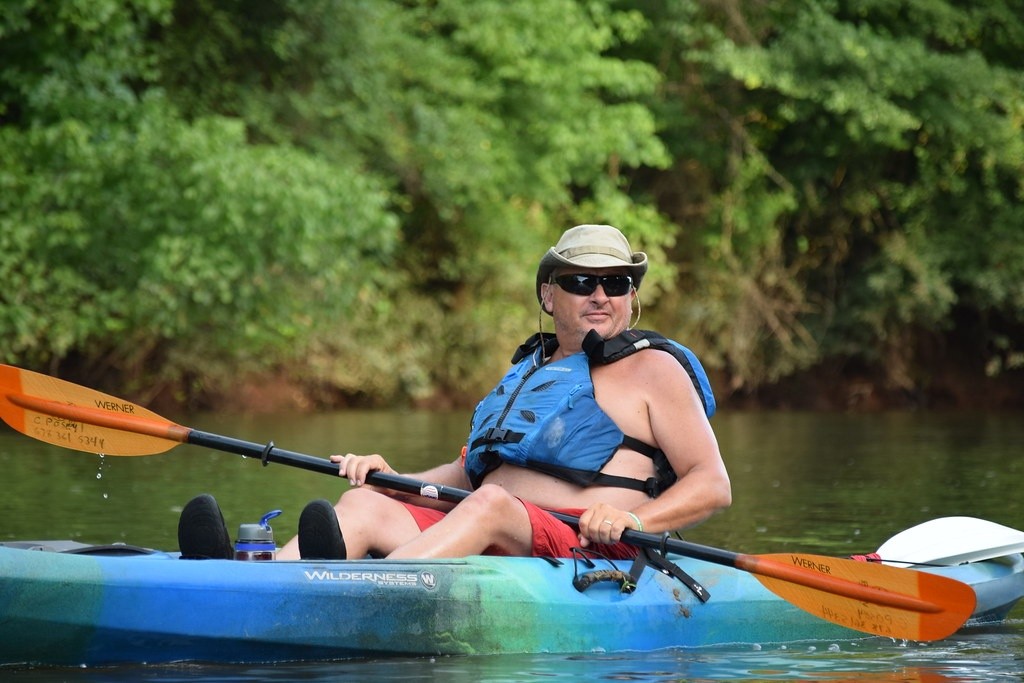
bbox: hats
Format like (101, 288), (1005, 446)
(535, 223), (648, 316)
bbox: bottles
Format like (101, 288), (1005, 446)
(233, 509), (282, 561)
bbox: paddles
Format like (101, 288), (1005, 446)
(0, 363), (977, 641)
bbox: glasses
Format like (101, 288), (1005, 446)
(550, 273), (634, 296)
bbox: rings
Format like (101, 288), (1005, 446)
(604, 520), (612, 525)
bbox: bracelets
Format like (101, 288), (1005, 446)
(626, 512), (643, 532)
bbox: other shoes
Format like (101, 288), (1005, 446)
(297, 498), (346, 561)
(178, 494), (235, 560)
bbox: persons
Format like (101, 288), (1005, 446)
(176, 223), (733, 564)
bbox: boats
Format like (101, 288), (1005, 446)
(0, 513), (1023, 660)
